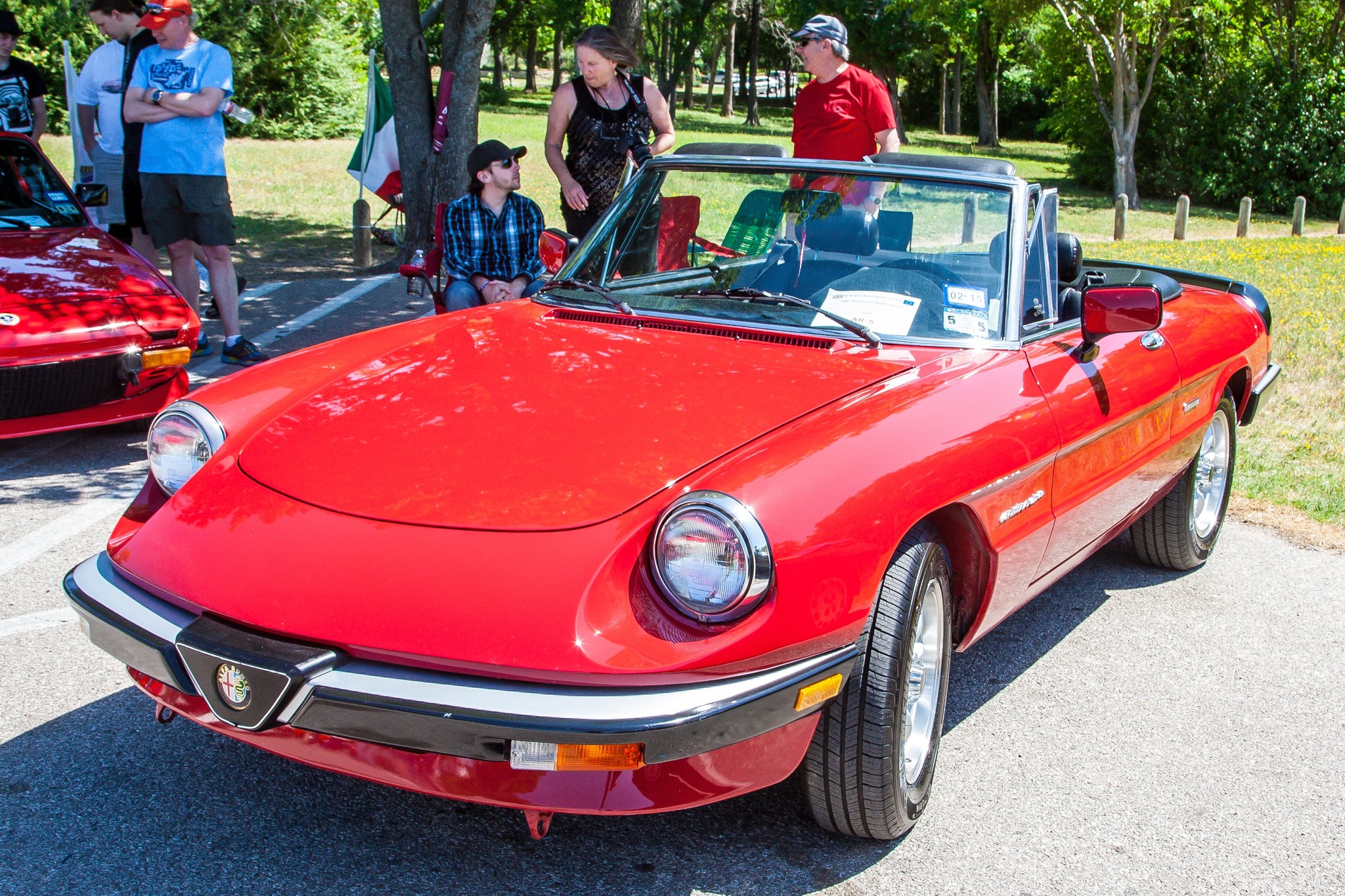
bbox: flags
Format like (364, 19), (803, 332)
(346, 57), (405, 214)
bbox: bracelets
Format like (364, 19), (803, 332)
(478, 279), (490, 293)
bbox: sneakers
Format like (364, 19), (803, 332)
(190, 332), (212, 358)
(205, 273), (246, 319)
(220, 337), (270, 366)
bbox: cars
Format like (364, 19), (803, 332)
(701, 69), (798, 94)
(0, 130), (203, 440)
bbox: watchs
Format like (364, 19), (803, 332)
(865, 192), (881, 205)
(151, 90), (164, 105)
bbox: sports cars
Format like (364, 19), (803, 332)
(61, 138), (1286, 841)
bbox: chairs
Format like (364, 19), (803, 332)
(398, 204), (456, 315)
(989, 229), (1085, 323)
(612, 190), (882, 301)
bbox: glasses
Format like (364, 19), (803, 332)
(800, 36), (824, 47)
(142, 4), (185, 15)
(487, 156), (519, 169)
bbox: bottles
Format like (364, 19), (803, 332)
(217, 98), (254, 124)
(407, 250), (426, 297)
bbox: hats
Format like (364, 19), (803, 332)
(466, 139), (526, 179)
(792, 13), (848, 46)
(135, 0), (193, 29)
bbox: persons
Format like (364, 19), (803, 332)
(73, 0), (270, 366)
(544, 24), (676, 282)
(444, 139), (547, 312)
(784, 14), (900, 242)
(0, 9), (49, 146)
